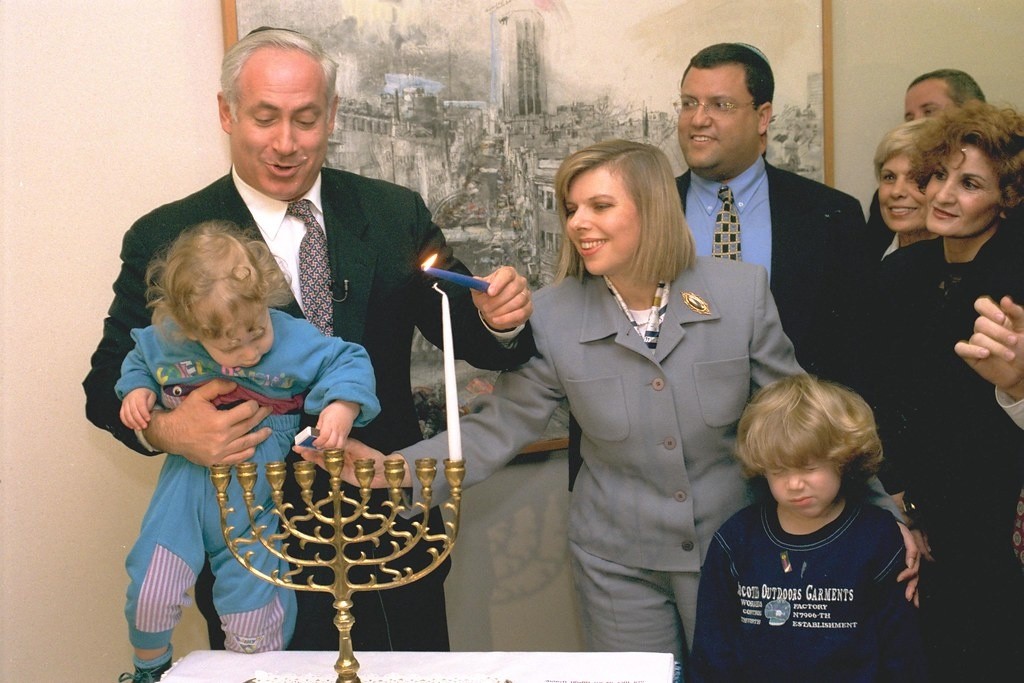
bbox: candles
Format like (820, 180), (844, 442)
(432, 280), (463, 463)
(419, 253), (490, 293)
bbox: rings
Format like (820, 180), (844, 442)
(521, 292), (528, 297)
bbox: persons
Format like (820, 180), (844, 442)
(862, 70), (1024, 683)
(689, 378), (927, 683)
(292, 137), (923, 663)
(566, 42), (892, 489)
(114, 224), (380, 683)
(83, 27), (534, 652)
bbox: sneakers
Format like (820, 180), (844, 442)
(118, 658), (172, 683)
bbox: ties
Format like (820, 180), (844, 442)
(712, 186), (742, 260)
(284, 197), (332, 339)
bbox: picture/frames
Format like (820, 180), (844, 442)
(219, 0), (834, 455)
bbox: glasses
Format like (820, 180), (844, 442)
(673, 96), (755, 120)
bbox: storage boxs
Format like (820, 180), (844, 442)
(294, 426), (319, 450)
